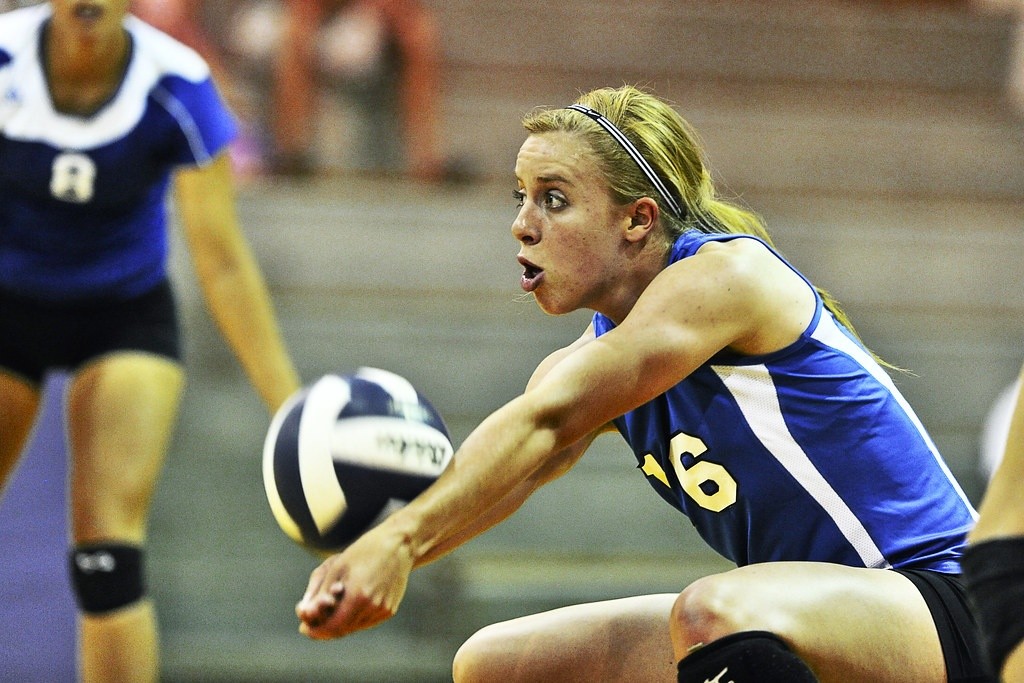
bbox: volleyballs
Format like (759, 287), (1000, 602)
(259, 366), (455, 553)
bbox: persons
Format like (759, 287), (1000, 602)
(0, 0), (302, 683)
(297, 86), (1000, 683)
